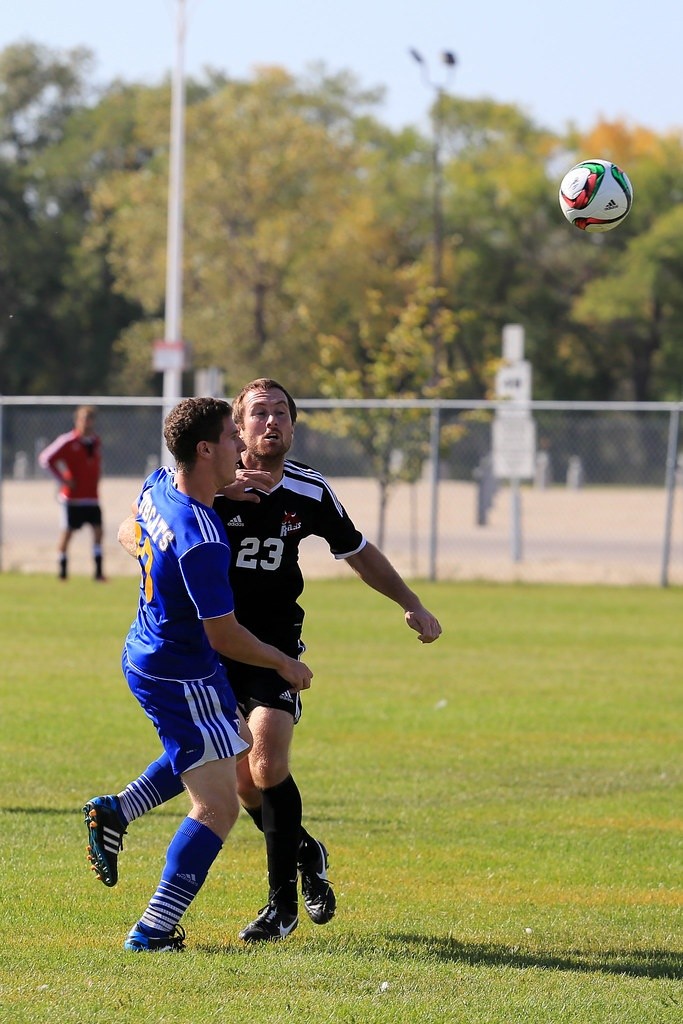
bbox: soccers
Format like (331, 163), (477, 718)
(557, 157), (635, 233)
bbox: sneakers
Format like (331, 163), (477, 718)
(298, 842), (336, 923)
(125, 921), (189, 952)
(80, 792), (126, 887)
(239, 901), (299, 942)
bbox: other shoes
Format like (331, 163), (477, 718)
(95, 574), (108, 581)
(60, 573), (66, 580)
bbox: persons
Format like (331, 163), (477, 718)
(81, 399), (314, 953)
(117, 378), (442, 940)
(39, 405), (108, 582)
